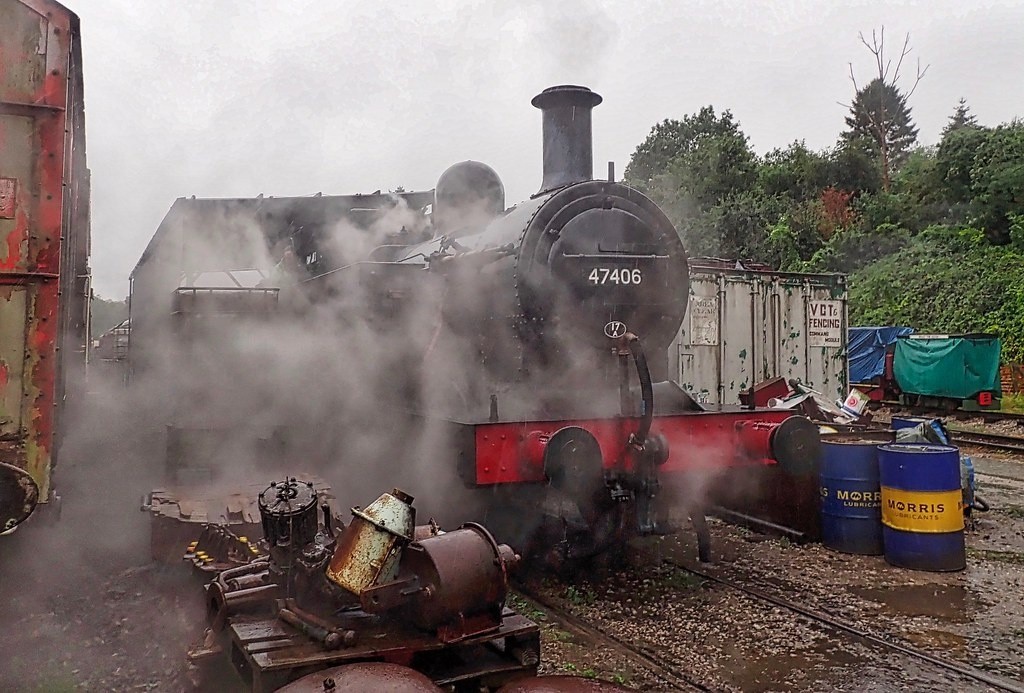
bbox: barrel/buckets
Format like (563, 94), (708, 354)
(818, 437), (968, 574)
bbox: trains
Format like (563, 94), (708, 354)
(93, 82), (819, 568)
